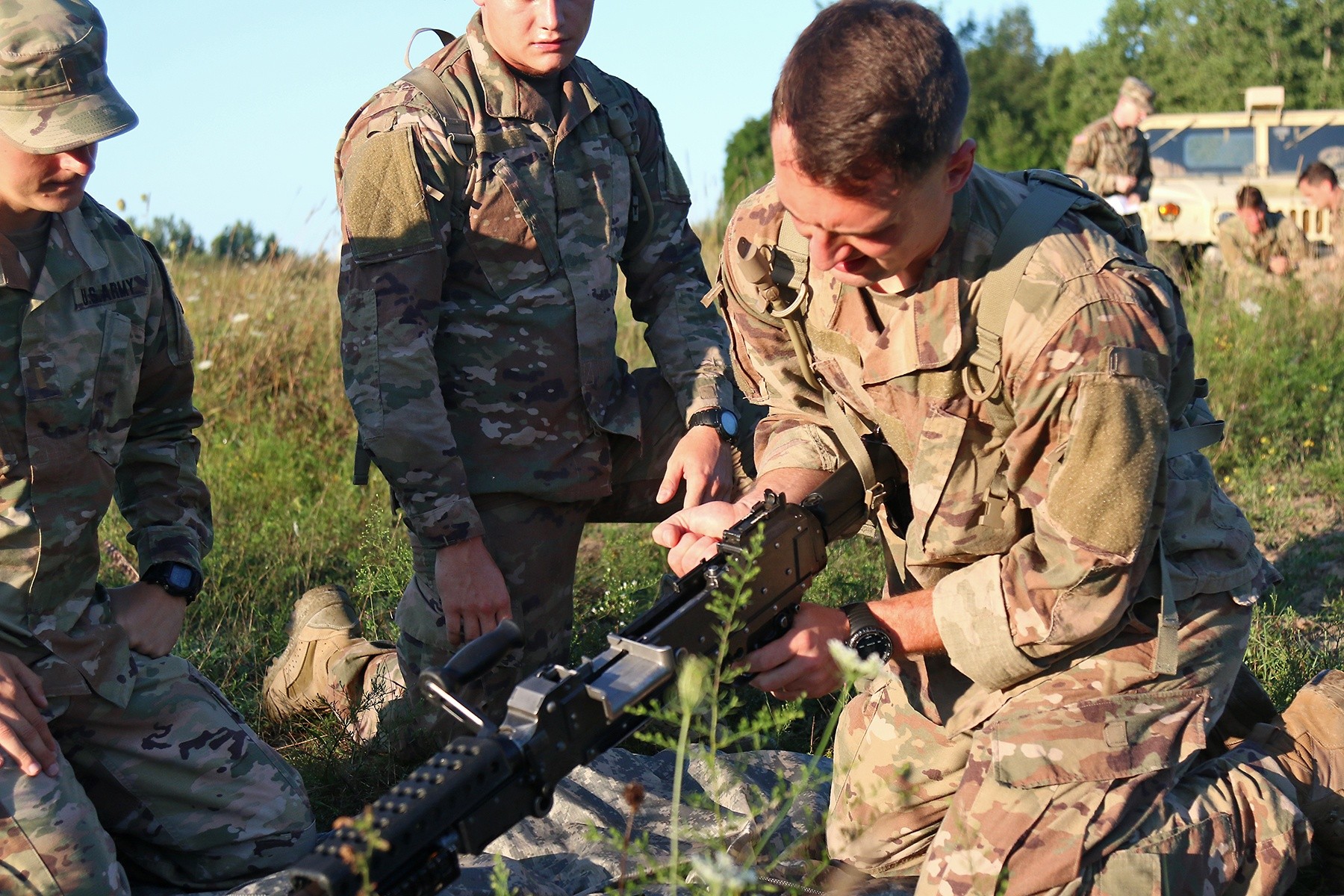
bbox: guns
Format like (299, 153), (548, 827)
(286, 432), (914, 896)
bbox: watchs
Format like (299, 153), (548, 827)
(139, 562), (203, 605)
(688, 407), (740, 443)
(839, 600), (894, 665)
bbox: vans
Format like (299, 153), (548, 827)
(1140, 86), (1344, 280)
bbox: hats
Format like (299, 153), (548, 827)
(0, 0), (139, 154)
(1120, 75), (1157, 114)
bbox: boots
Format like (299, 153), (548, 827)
(1188, 661), (1278, 769)
(260, 585), (368, 718)
(1240, 666), (1344, 896)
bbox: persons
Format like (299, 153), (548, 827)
(1295, 160), (1344, 292)
(261, 0), (736, 774)
(0, 0), (323, 895)
(1065, 76), (1154, 261)
(1218, 186), (1311, 313)
(653, 0), (1344, 896)
